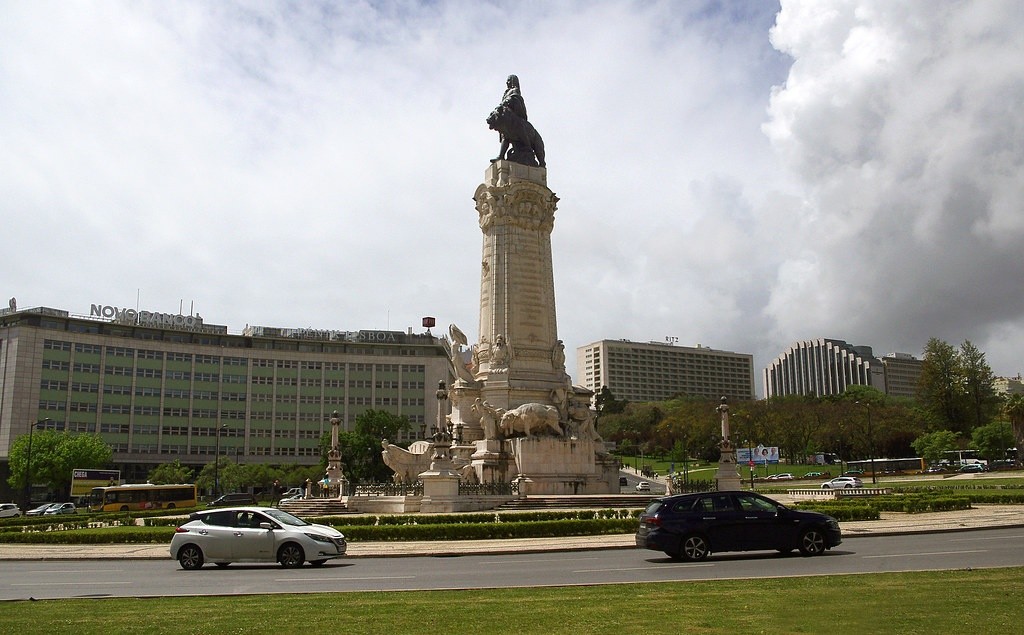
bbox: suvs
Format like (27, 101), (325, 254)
(205, 493), (259, 508)
(634, 490), (844, 562)
(168, 506), (348, 569)
(0, 504), (21, 518)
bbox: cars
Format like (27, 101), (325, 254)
(799, 472), (822, 479)
(838, 471), (865, 478)
(957, 464), (985, 473)
(619, 476), (627, 486)
(924, 466), (942, 472)
(26, 503), (55, 517)
(764, 472), (795, 479)
(821, 477), (863, 489)
(277, 487), (313, 506)
(636, 482), (651, 491)
(44, 502), (78, 516)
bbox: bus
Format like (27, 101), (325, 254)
(938, 448), (1018, 467)
(87, 484), (198, 515)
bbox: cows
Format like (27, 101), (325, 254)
(498, 403), (564, 439)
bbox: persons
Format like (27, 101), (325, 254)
(770, 448), (778, 460)
(324, 478), (330, 499)
(107, 477), (116, 487)
(752, 448), (762, 461)
(269, 480), (283, 509)
(486, 75), (547, 167)
(761, 449), (770, 460)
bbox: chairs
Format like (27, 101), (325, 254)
(239, 513), (261, 528)
(721, 497), (743, 511)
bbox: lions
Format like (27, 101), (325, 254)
(485, 103), (547, 168)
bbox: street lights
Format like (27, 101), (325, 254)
(855, 401), (876, 484)
(733, 414), (754, 488)
(213, 423), (230, 500)
(23, 418), (54, 516)
